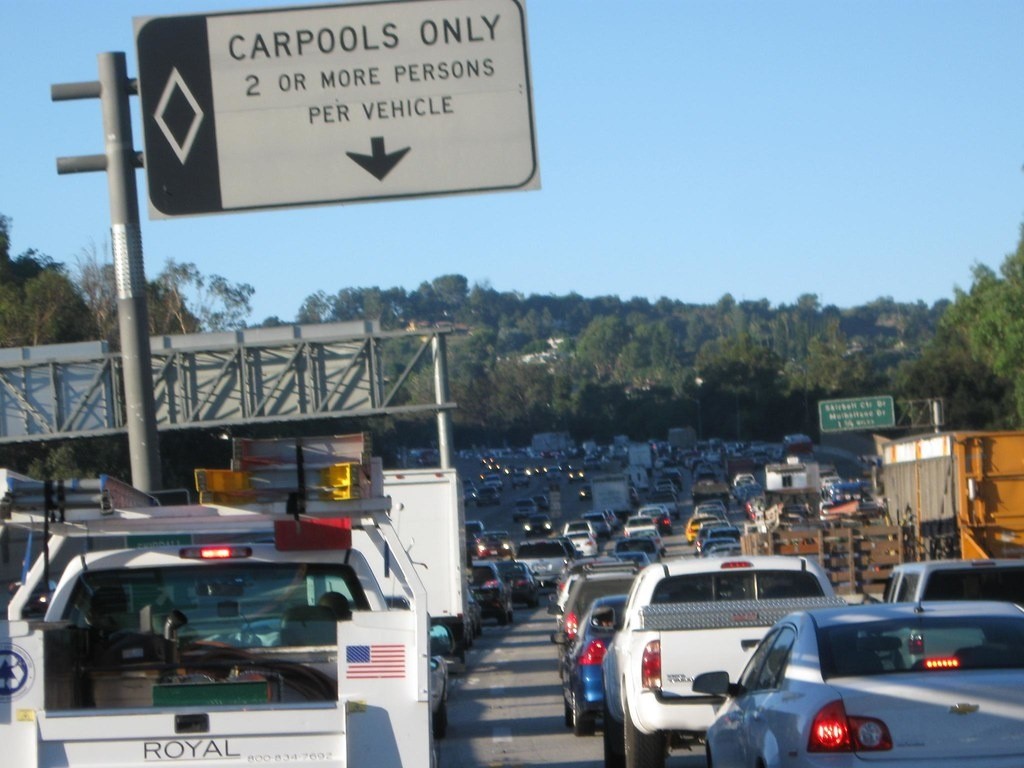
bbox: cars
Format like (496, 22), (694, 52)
(551, 594), (628, 737)
(692, 602), (1024, 768)
(388, 424), (891, 645)
(427, 612), (456, 740)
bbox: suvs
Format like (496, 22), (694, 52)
(600, 555), (846, 768)
(883, 560), (1024, 610)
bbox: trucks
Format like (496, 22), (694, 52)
(870, 429), (1024, 564)
(0, 321), (472, 767)
